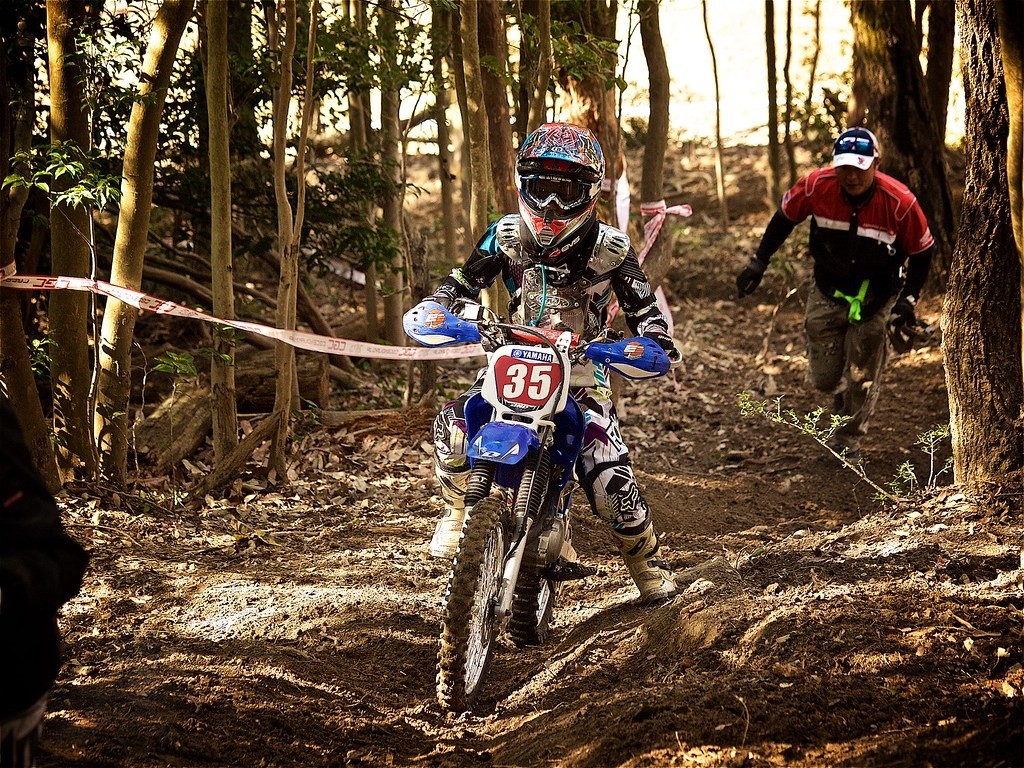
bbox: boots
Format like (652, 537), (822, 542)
(430, 465), (473, 561)
(608, 521), (679, 607)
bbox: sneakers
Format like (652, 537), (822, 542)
(837, 450), (863, 471)
(832, 373), (850, 412)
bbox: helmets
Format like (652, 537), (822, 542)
(513, 123), (607, 248)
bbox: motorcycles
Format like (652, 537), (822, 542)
(401, 297), (671, 714)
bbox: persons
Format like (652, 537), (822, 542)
(0, 389), (91, 766)
(400, 123), (682, 605)
(736, 127), (939, 463)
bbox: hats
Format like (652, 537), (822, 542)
(830, 126), (879, 171)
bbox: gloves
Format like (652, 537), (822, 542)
(736, 257), (767, 297)
(891, 297), (917, 328)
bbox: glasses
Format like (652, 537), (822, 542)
(834, 142), (879, 155)
(514, 168), (601, 210)
